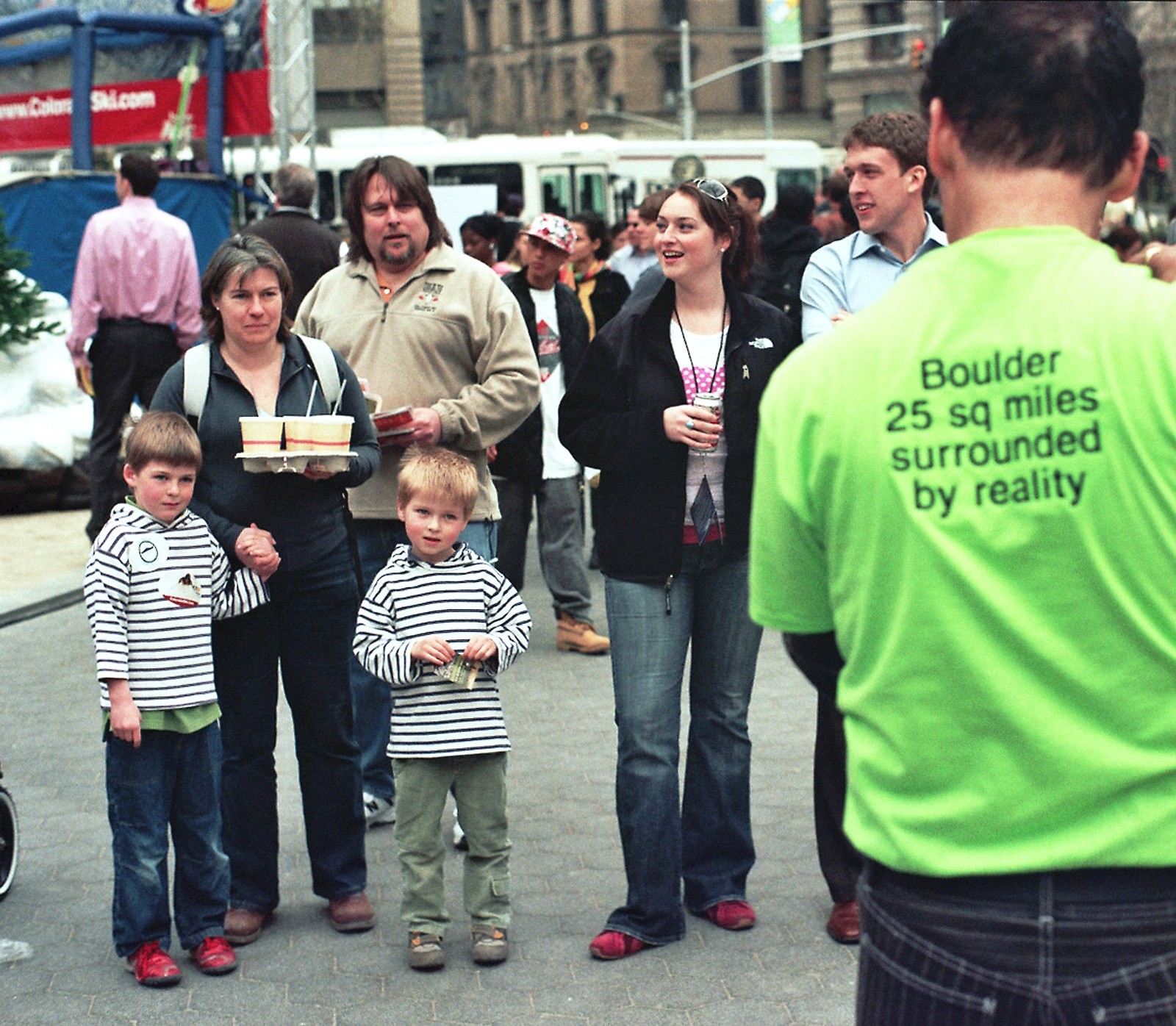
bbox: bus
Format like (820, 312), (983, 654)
(224, 125), (847, 263)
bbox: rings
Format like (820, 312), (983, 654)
(686, 418), (695, 430)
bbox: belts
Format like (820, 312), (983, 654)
(867, 866), (1175, 903)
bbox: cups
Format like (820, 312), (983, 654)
(239, 417), (284, 453)
(283, 415), (317, 451)
(311, 415), (354, 451)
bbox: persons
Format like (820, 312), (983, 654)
(496, 216), (611, 652)
(152, 235), (383, 947)
(804, 111), (954, 947)
(67, 151), (204, 547)
(352, 448), (531, 968)
(557, 182), (800, 959)
(459, 141), (1176, 342)
(82, 408), (278, 989)
(234, 164), (350, 331)
(746, 0), (1176, 1023)
(288, 156), (543, 851)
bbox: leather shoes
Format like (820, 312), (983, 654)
(829, 898), (860, 944)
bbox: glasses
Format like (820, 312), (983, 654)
(688, 177), (730, 209)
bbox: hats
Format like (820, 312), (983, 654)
(519, 213), (577, 254)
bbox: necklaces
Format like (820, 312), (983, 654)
(671, 298), (728, 544)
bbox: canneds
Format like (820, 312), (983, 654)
(692, 392), (722, 452)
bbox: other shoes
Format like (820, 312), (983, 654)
(224, 907), (268, 943)
(327, 889), (376, 931)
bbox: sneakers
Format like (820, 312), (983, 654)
(470, 923), (508, 963)
(191, 936), (236, 974)
(407, 930), (445, 968)
(452, 805), (470, 852)
(124, 939), (182, 986)
(709, 899), (755, 931)
(556, 611), (611, 653)
(589, 930), (648, 960)
(362, 791), (397, 829)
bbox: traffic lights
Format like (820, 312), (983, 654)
(911, 38), (927, 71)
(579, 118), (590, 134)
